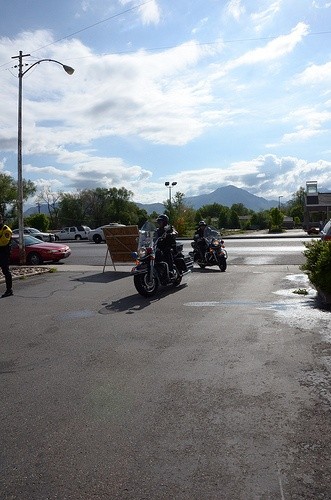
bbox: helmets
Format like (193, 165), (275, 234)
(199, 221), (206, 227)
(156, 214), (169, 225)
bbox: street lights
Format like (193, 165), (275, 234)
(17, 59), (75, 263)
(164, 181), (177, 206)
(279, 196), (284, 209)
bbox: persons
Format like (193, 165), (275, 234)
(153, 214), (178, 278)
(193, 221), (221, 263)
(0, 214), (13, 298)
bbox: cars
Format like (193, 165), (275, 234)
(9, 234), (72, 264)
(54, 226), (87, 240)
(87, 223), (147, 244)
(13, 227), (54, 241)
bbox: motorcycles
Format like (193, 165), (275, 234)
(189, 225), (228, 271)
(131, 218), (188, 297)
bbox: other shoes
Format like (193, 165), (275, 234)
(2, 289), (13, 297)
(169, 271), (174, 277)
